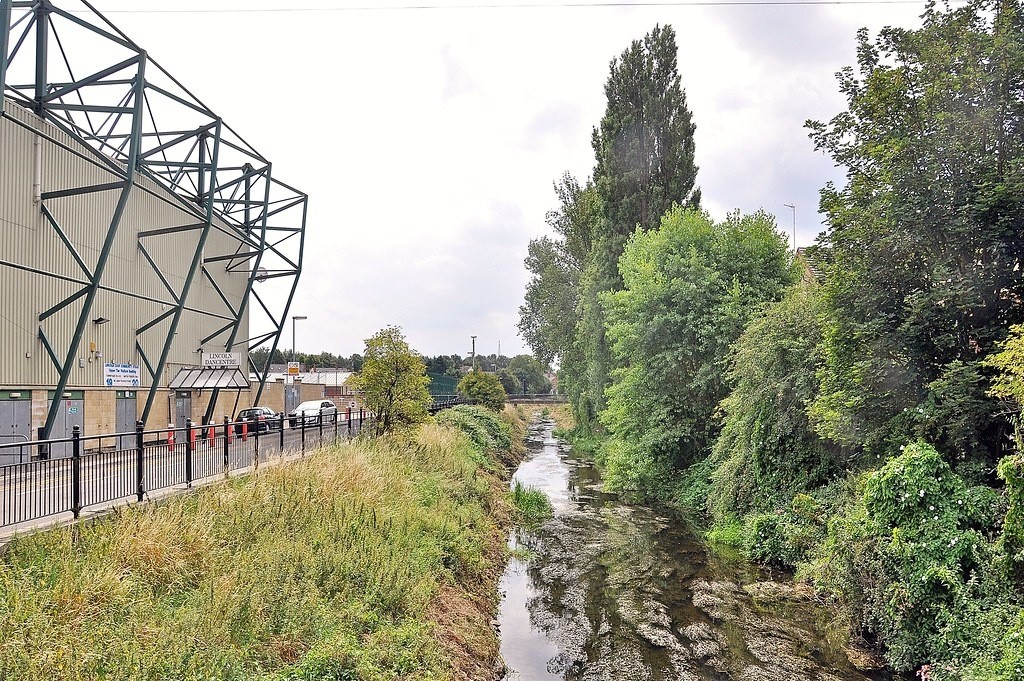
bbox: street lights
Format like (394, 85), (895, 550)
(291, 315), (308, 409)
(470, 335), (478, 368)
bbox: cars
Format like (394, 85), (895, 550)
(292, 399), (338, 428)
(234, 406), (281, 439)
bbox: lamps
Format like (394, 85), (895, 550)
(92, 317), (110, 324)
